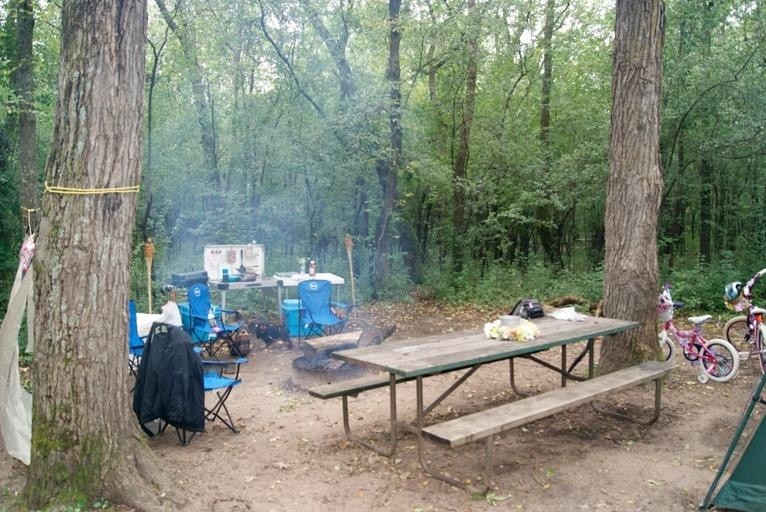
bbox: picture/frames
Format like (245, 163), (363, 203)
(330, 312), (640, 469)
(214, 273), (345, 323)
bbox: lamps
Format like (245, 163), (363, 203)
(296, 278), (351, 350)
(126, 283), (248, 447)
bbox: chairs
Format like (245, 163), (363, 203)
(126, 283), (248, 447)
(296, 278), (351, 350)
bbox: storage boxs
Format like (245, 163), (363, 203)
(281, 299), (326, 338)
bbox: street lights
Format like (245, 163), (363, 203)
(517, 299), (544, 320)
(231, 330), (250, 356)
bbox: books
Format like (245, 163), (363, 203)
(281, 299), (326, 338)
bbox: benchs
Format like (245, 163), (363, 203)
(308, 347), (547, 458)
(421, 360), (679, 495)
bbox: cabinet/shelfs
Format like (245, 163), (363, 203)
(309, 260), (316, 276)
(222, 269), (228, 281)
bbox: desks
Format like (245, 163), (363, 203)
(214, 273), (345, 323)
(330, 312), (640, 469)
